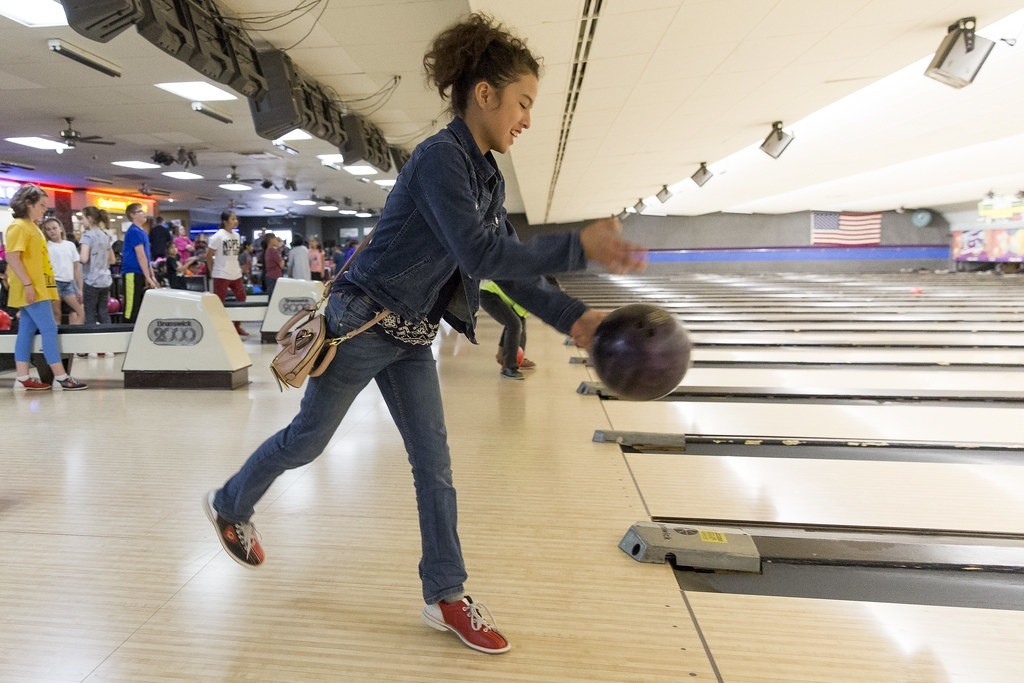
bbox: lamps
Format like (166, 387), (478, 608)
(759, 122), (793, 158)
(179, 147), (198, 168)
(633, 198), (646, 213)
(656, 185), (672, 203)
(286, 179), (376, 213)
(691, 162), (712, 186)
(924, 17), (995, 87)
(618, 208), (630, 220)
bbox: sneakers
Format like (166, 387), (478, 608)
(422, 594), (511, 654)
(203, 490), (265, 568)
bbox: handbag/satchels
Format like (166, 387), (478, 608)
(270, 305), (337, 390)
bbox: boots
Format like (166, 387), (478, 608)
(495, 346), (503, 365)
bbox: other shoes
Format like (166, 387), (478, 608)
(521, 359), (537, 366)
(500, 370), (524, 379)
(51, 376), (88, 390)
(14, 377), (51, 391)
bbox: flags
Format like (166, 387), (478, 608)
(810, 211), (882, 246)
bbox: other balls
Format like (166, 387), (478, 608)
(589, 303), (693, 403)
(0, 310), (13, 330)
(244, 283), (254, 294)
(107, 296), (120, 313)
(516, 346), (524, 365)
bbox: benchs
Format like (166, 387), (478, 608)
(0, 322), (134, 352)
(220, 293), (267, 321)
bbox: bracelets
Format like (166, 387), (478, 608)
(23, 282), (33, 287)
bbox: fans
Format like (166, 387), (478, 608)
(205, 166), (261, 184)
(44, 117), (116, 150)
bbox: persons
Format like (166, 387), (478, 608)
(202, 13), (650, 653)
(5, 184), (89, 392)
(480, 270), (539, 381)
(0, 203), (360, 336)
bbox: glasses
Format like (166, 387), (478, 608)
(131, 210), (143, 213)
